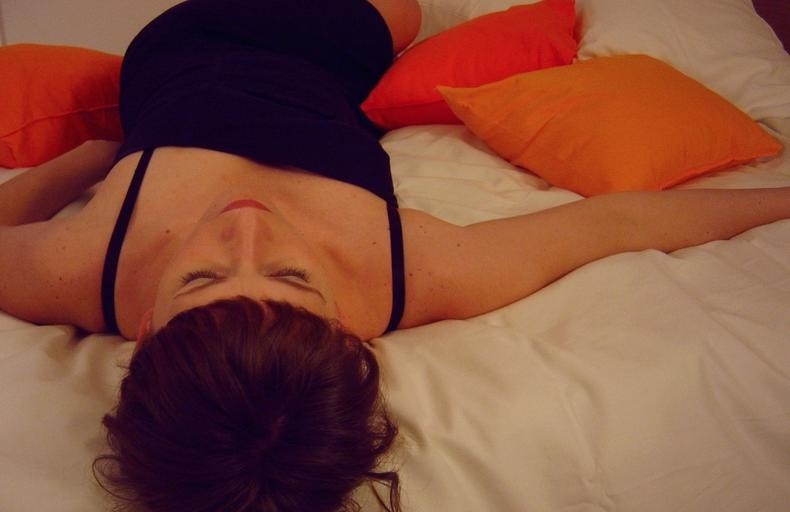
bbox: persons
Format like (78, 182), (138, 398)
(0, 0), (790, 512)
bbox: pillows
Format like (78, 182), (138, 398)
(0, 42), (123, 169)
(440, 51), (785, 200)
(363, 1), (583, 130)
